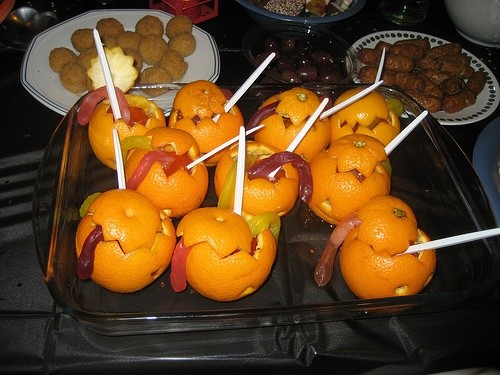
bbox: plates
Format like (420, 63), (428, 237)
(31, 83), (500, 338)
(345, 30), (500, 126)
(0, 0), (14, 24)
(236, 0), (366, 24)
(20, 8), (221, 118)
(472, 116), (500, 228)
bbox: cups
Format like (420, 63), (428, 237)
(382, 0), (429, 27)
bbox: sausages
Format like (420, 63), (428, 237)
(356, 38), (486, 112)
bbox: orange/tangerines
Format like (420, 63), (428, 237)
(76, 80), (438, 304)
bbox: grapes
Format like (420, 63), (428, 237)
(257, 38), (347, 83)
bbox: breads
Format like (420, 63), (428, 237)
(49, 15), (198, 96)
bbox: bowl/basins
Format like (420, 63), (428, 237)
(444, 0), (499, 48)
(241, 23), (359, 87)
